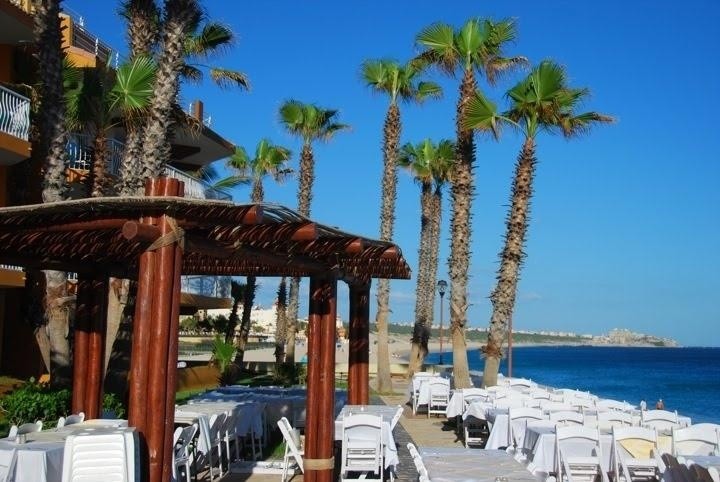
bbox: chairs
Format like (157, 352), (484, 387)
(1, 382), (404, 482)
(407, 372), (720, 482)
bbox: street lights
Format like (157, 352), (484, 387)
(437, 279), (448, 365)
